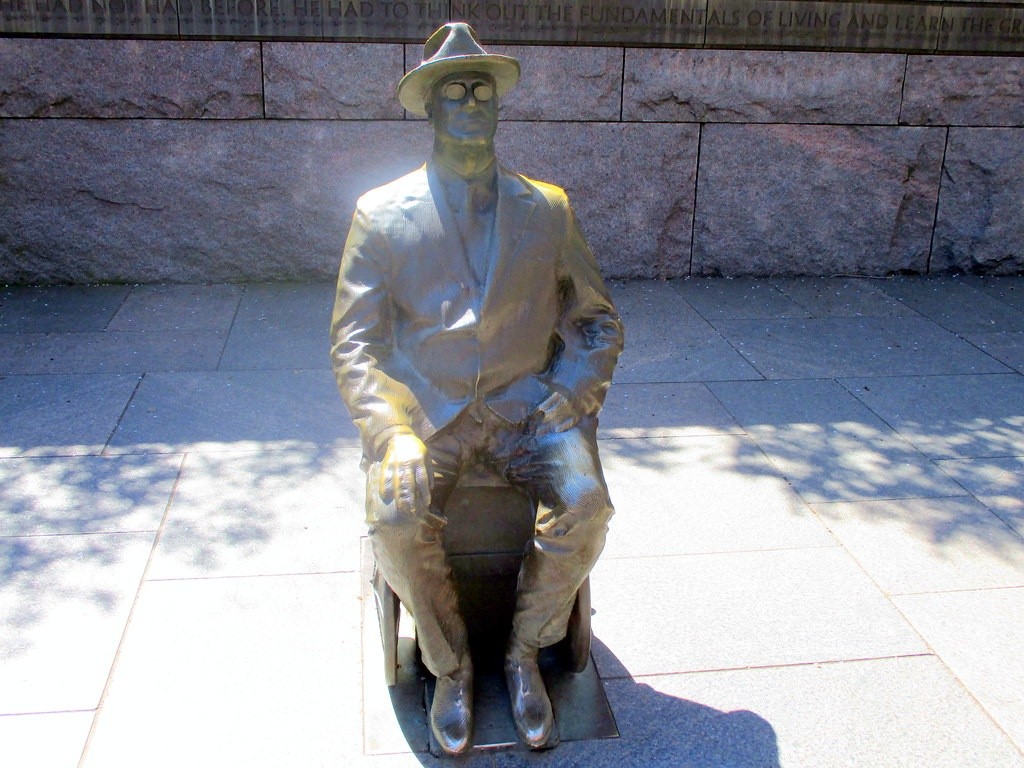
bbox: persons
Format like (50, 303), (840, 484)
(329, 22), (625, 757)
